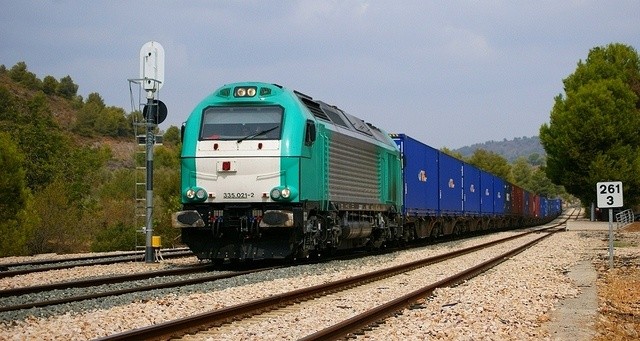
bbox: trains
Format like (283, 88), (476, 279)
(168, 81), (563, 263)
